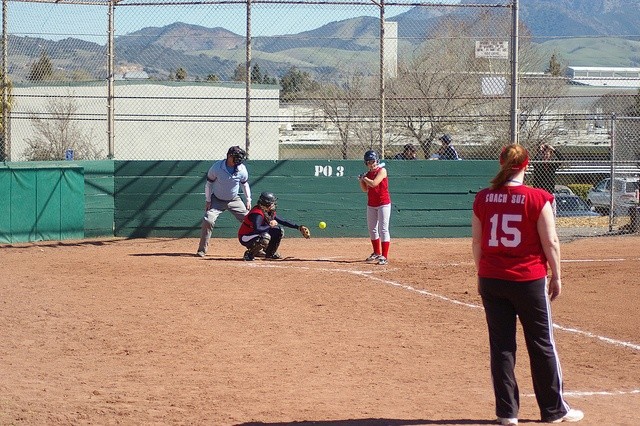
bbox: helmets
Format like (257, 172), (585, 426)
(258, 192), (278, 205)
(364, 150), (378, 163)
(229, 146), (246, 166)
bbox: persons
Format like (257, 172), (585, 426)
(472, 143), (584, 426)
(359, 150), (392, 265)
(196, 145), (266, 257)
(430, 133), (459, 160)
(531, 143), (565, 229)
(392, 143), (419, 160)
(237, 192), (311, 261)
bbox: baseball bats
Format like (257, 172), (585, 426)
(359, 162), (385, 180)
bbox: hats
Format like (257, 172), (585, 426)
(439, 135), (452, 141)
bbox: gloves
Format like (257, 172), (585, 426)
(357, 174), (364, 182)
(361, 173), (367, 179)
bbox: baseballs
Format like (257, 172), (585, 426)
(319, 222), (326, 229)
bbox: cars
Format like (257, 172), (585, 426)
(555, 195), (599, 217)
(555, 185), (574, 195)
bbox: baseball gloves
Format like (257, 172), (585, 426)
(299, 225), (310, 239)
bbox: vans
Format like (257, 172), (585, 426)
(587, 177), (639, 216)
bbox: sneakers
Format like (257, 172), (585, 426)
(256, 251), (266, 256)
(265, 252), (280, 259)
(197, 251), (205, 256)
(366, 253), (381, 261)
(377, 256), (387, 265)
(496, 417), (518, 426)
(244, 250), (254, 261)
(548, 409), (584, 424)
